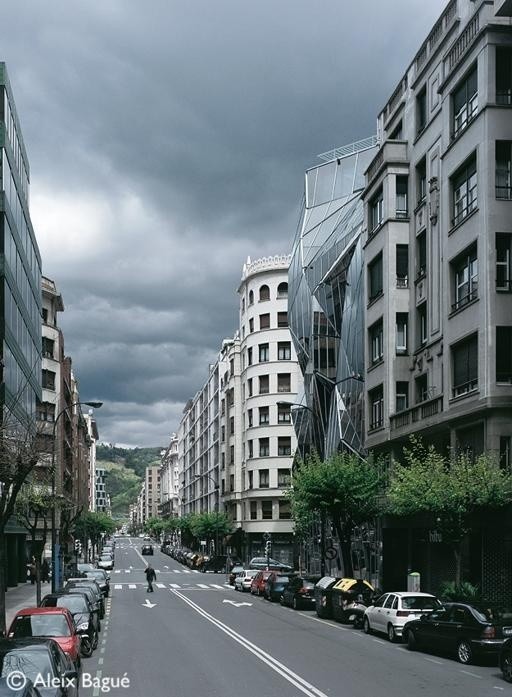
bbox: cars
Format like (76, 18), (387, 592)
(113, 527), (132, 538)
(143, 534), (151, 541)
(0, 536), (115, 695)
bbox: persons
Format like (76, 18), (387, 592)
(41, 559), (50, 582)
(25, 557), (37, 584)
(144, 563), (157, 592)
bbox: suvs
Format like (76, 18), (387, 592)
(141, 544), (154, 555)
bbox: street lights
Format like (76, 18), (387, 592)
(163, 491), (183, 520)
(195, 472), (220, 571)
(93, 474), (107, 513)
(274, 400), (327, 577)
(49, 401), (101, 593)
